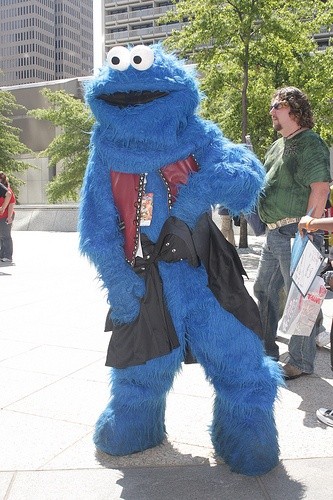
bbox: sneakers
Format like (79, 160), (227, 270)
(281, 363), (308, 380)
(316, 408), (333, 427)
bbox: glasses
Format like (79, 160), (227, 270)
(268, 101), (290, 112)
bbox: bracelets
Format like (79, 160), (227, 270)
(306, 218), (320, 232)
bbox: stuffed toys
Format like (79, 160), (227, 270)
(78, 44), (288, 474)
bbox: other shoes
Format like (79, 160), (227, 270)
(1, 257), (12, 262)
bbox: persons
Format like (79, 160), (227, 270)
(280, 285), (330, 348)
(254, 85), (333, 380)
(0, 171), (16, 262)
(217, 204), (238, 248)
(297, 215), (333, 428)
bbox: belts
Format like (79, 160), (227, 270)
(267, 217), (302, 230)
